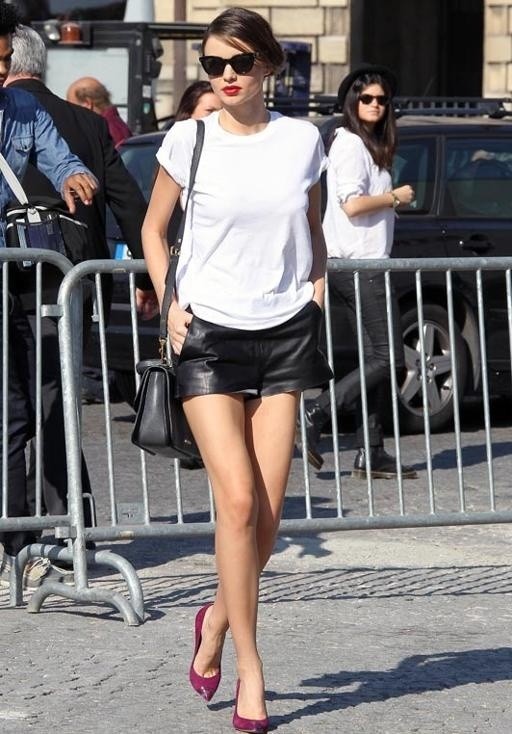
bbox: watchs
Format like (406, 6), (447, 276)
(388, 192), (401, 209)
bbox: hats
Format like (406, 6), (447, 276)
(339, 64), (398, 107)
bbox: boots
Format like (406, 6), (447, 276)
(295, 401), (329, 469)
(353, 446), (416, 480)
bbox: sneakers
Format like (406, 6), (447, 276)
(0, 549), (74, 592)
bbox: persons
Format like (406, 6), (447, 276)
(66, 76), (133, 148)
(139, 8), (326, 734)
(3, 25), (160, 551)
(169, 80), (227, 120)
(1, 19), (101, 590)
(294, 72), (417, 478)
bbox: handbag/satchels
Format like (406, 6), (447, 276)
(133, 366), (205, 470)
(5, 202), (88, 290)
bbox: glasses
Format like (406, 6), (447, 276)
(360, 94), (387, 105)
(199, 52), (264, 75)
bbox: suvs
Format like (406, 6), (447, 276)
(27, 12), (315, 282)
(65, 87), (511, 435)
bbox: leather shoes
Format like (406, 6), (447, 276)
(41, 535), (95, 570)
(191, 605), (221, 700)
(233, 678), (269, 733)
